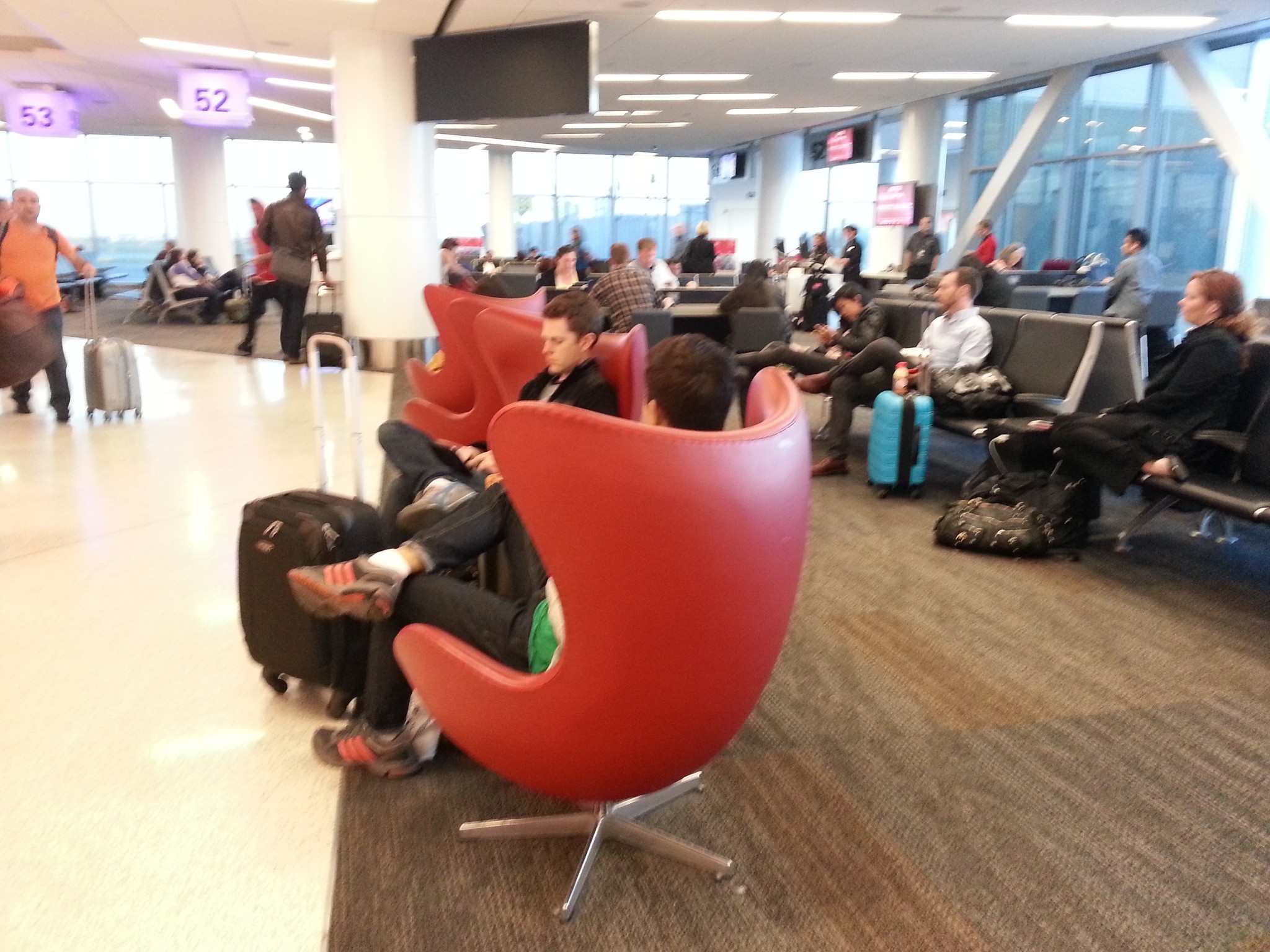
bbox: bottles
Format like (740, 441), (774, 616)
(893, 361), (909, 394)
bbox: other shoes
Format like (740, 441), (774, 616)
(51, 403), (70, 422)
(17, 402), (29, 414)
(237, 342), (253, 356)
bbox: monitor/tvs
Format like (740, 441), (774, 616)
(718, 151), (736, 179)
(826, 127), (853, 163)
(875, 182), (916, 226)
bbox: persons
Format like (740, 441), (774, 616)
(679, 220), (715, 274)
(720, 260), (781, 313)
(839, 226), (862, 283)
(1099, 226), (1165, 328)
(286, 333), (738, 779)
(437, 222), (688, 329)
(806, 231), (826, 263)
(898, 215), (940, 279)
(794, 265), (993, 477)
(137, 170), (337, 364)
(0, 187), (99, 424)
(732, 281), (888, 382)
(375, 289), (619, 563)
(971, 218), (997, 267)
(1047, 269), (1252, 549)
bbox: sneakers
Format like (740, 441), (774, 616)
(286, 553), (402, 626)
(396, 480), (479, 534)
(312, 715), (420, 779)
(793, 371), (831, 395)
(812, 456), (847, 476)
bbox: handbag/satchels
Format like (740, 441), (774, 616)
(1041, 252), (1115, 283)
(969, 470), (1094, 550)
(789, 272), (830, 332)
(224, 298), (253, 324)
(931, 364), (1015, 421)
(933, 497), (1058, 557)
(0, 293), (59, 389)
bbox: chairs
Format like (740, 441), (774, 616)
(56, 249), (232, 327)
(392, 255), (1270, 921)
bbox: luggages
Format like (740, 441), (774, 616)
(238, 489), (380, 720)
(84, 269), (141, 422)
(301, 282), (344, 367)
(867, 390), (933, 499)
